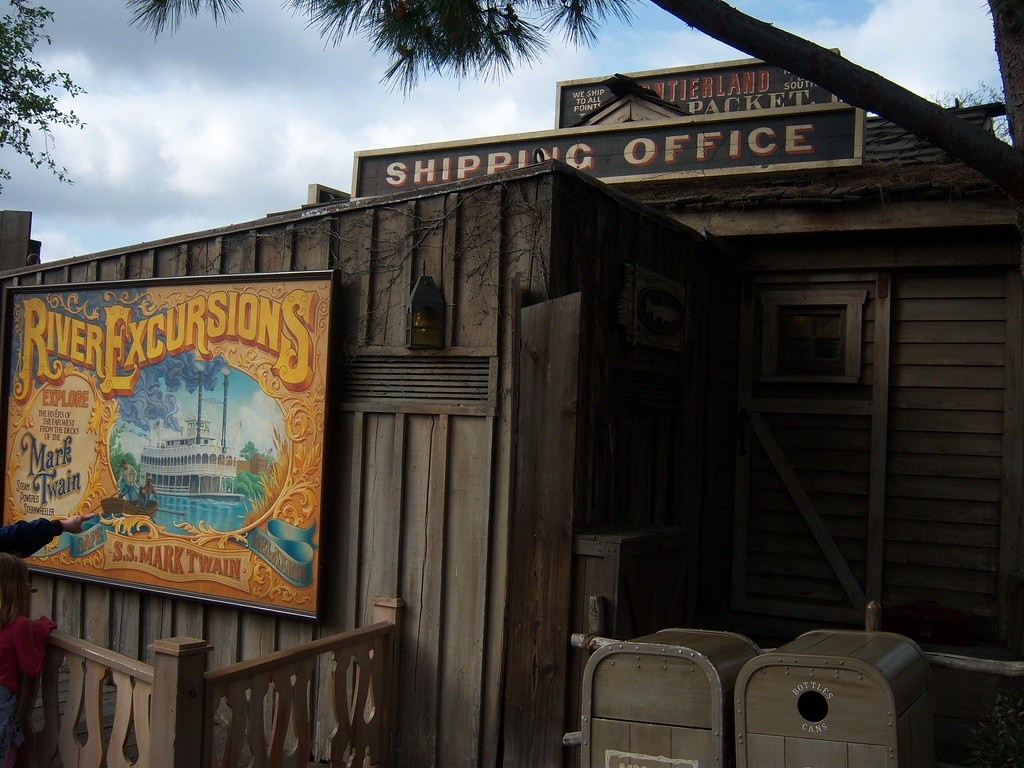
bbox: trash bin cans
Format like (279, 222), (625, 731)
(579, 628), (765, 768)
(734, 628), (934, 768)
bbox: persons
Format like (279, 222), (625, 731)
(0, 512), (95, 558)
(0, 552), (57, 767)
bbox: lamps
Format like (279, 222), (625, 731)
(403, 259), (447, 350)
